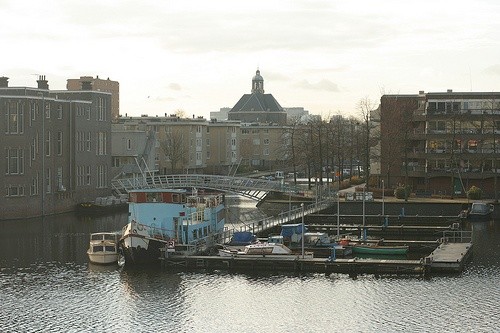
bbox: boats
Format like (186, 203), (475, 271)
(86, 230), (118, 264)
(336, 225), (410, 257)
(119, 185), (226, 268)
(74, 193), (130, 216)
(468, 200), (494, 221)
(230, 224), (353, 258)
(217, 240), (313, 261)
